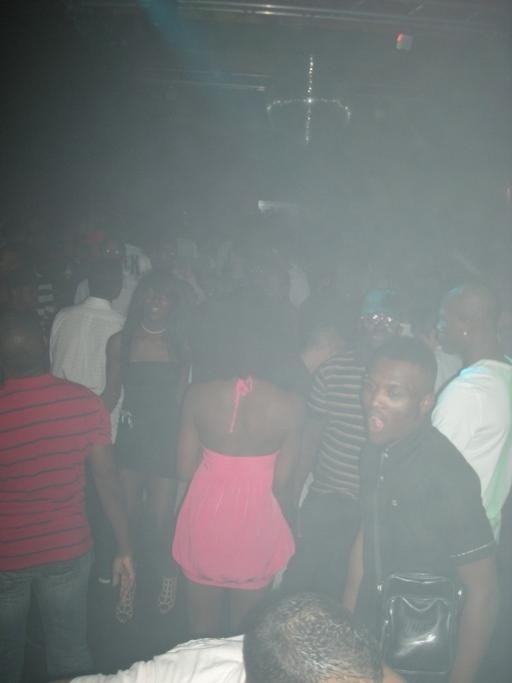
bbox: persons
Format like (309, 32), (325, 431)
(1, 217), (510, 682)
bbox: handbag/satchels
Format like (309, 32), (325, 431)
(374, 573), (463, 676)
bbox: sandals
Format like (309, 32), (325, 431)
(158, 572), (178, 614)
(114, 576), (136, 623)
